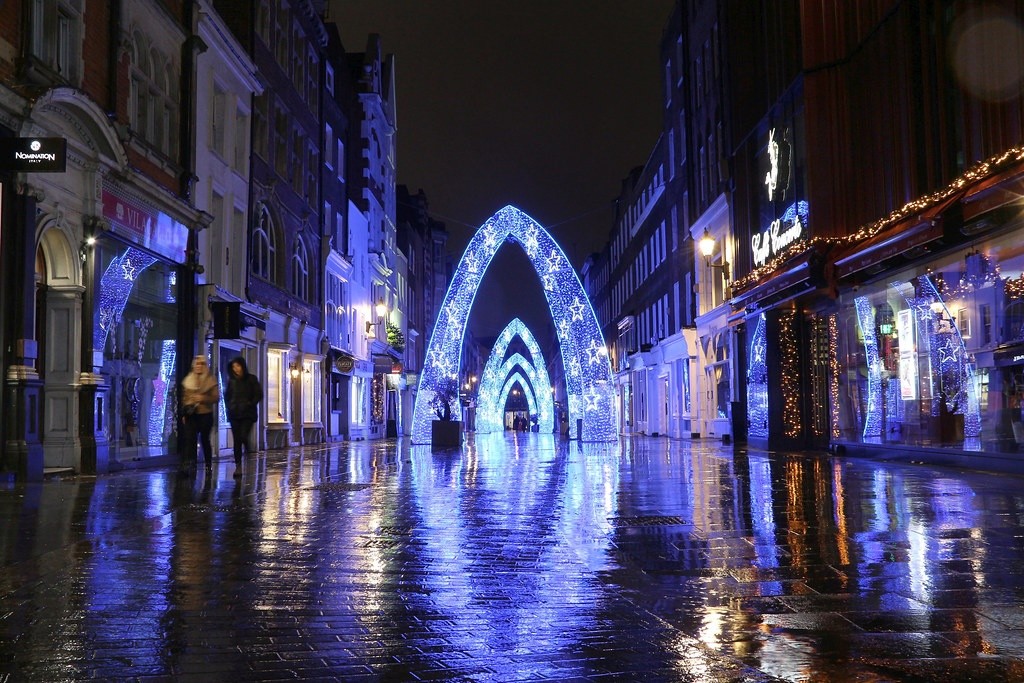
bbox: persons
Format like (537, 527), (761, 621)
(521, 417), (527, 433)
(174, 355), (219, 479)
(514, 416), (520, 431)
(224, 356), (263, 478)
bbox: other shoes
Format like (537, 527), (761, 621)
(188, 466), (197, 473)
(233, 464), (243, 476)
(205, 463), (212, 474)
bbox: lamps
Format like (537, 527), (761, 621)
(700, 231), (730, 279)
(289, 363), (300, 379)
(302, 366), (311, 384)
(366, 297), (387, 333)
